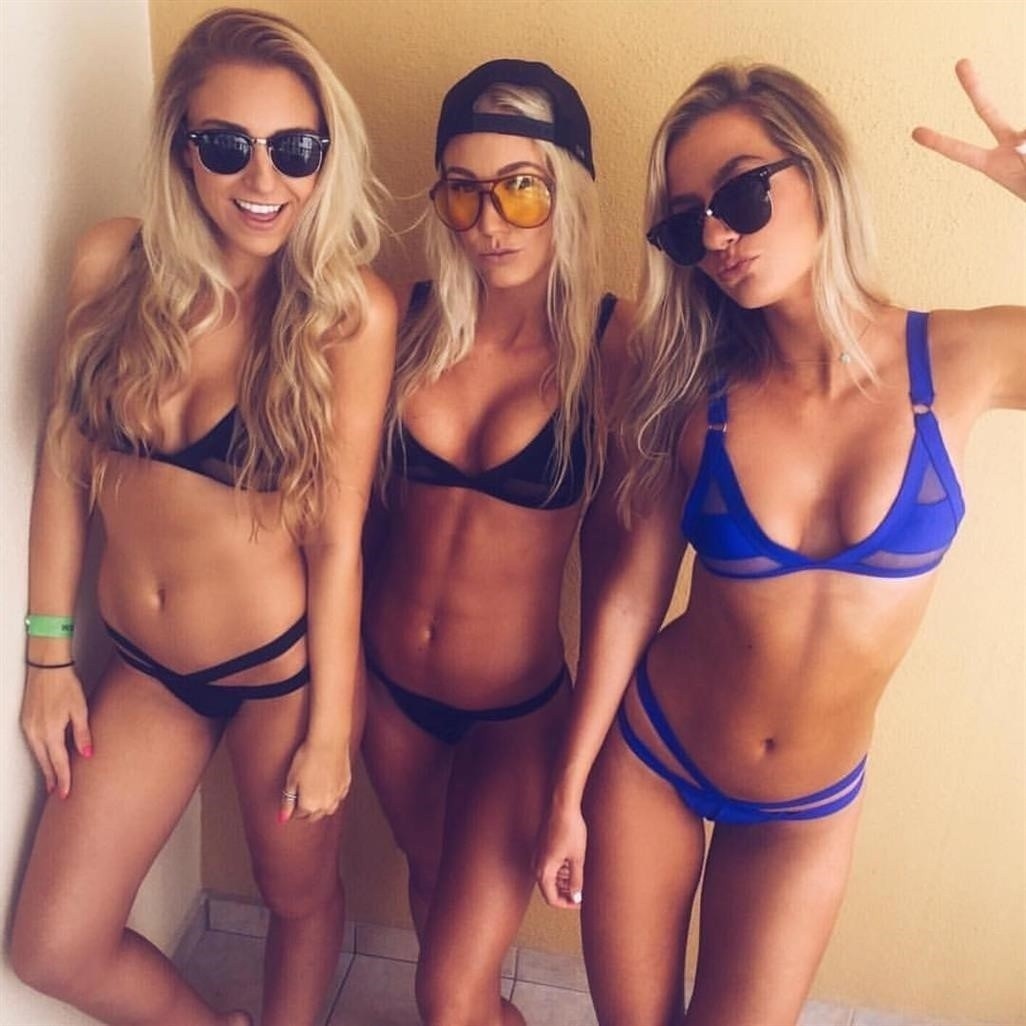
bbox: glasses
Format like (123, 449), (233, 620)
(430, 173), (552, 231)
(645, 158), (797, 269)
(187, 129), (331, 179)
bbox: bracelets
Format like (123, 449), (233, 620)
(26, 658), (75, 669)
(26, 613), (75, 639)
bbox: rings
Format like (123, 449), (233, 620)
(281, 789), (297, 800)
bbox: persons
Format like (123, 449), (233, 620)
(369, 61), (639, 1026)
(535, 58), (1026, 1026)
(10, 9), (396, 1026)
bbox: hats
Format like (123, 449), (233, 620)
(435, 59), (595, 182)
